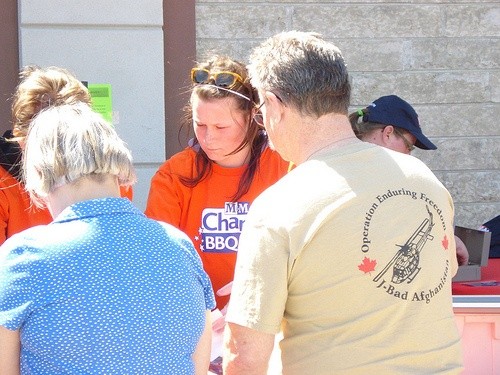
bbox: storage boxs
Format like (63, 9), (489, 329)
(451, 226), (491, 281)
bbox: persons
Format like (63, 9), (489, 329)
(143, 55), (296, 375)
(348, 94), (469, 265)
(0, 101), (216, 375)
(223, 31), (463, 375)
(0, 63), (133, 246)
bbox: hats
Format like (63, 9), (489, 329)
(364, 95), (437, 150)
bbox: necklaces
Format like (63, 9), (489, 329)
(302, 135), (356, 164)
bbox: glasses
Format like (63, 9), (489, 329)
(379, 125), (418, 152)
(190, 67), (250, 90)
(252, 93), (284, 127)
